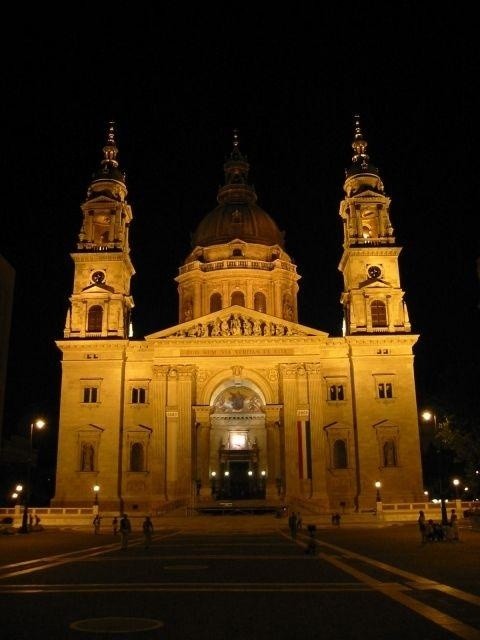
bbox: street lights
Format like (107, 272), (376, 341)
(92, 485), (101, 506)
(20, 417), (47, 534)
(421, 405), (449, 526)
(375, 481), (382, 502)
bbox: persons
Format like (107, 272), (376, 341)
(296, 511), (303, 529)
(119, 513), (133, 552)
(34, 515), (41, 527)
(288, 511), (298, 542)
(142, 516), (155, 551)
(417, 507), (458, 544)
(98, 516), (103, 534)
(331, 512), (335, 527)
(92, 513), (99, 535)
(112, 516), (118, 536)
(334, 513), (342, 529)
(28, 514), (34, 526)
